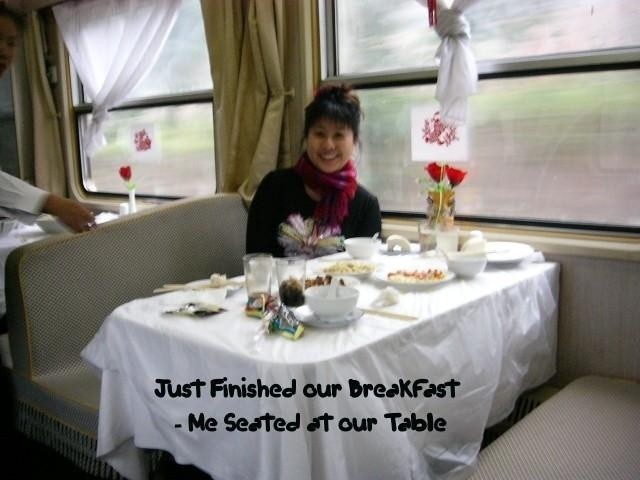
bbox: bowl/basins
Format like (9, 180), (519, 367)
(302, 285), (360, 323)
(34, 219), (65, 234)
(445, 253), (486, 279)
(344, 237), (381, 260)
(184, 280), (228, 304)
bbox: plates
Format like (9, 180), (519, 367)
(485, 241), (534, 266)
(312, 260), (382, 281)
(292, 305), (362, 329)
(374, 269), (455, 292)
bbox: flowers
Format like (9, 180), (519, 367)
(114, 165), (136, 188)
(418, 160), (470, 231)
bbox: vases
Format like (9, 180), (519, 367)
(415, 227), (462, 255)
(128, 189), (137, 216)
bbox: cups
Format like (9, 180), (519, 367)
(242, 252), (274, 306)
(274, 257), (308, 308)
(120, 202), (129, 217)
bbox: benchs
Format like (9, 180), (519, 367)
(1, 183), (258, 479)
(441, 364), (639, 479)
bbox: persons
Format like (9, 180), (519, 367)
(246, 81), (382, 257)
(0, 7), (97, 234)
